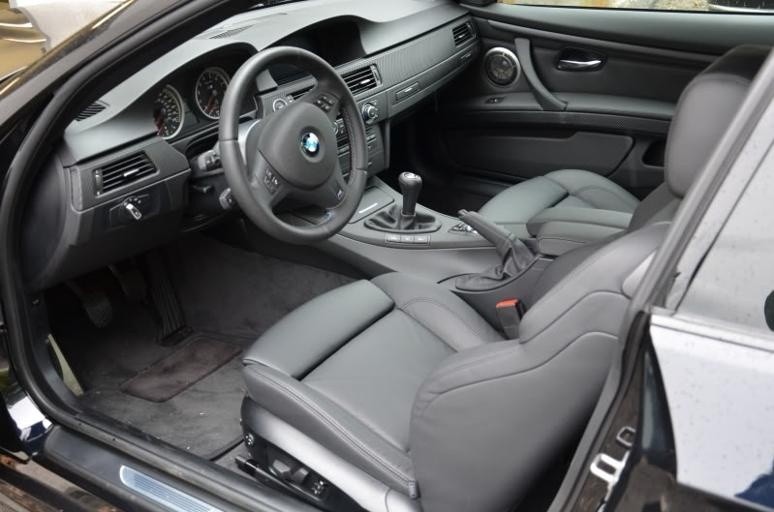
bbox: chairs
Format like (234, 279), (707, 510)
(241, 74), (751, 512)
(477, 43), (770, 242)
